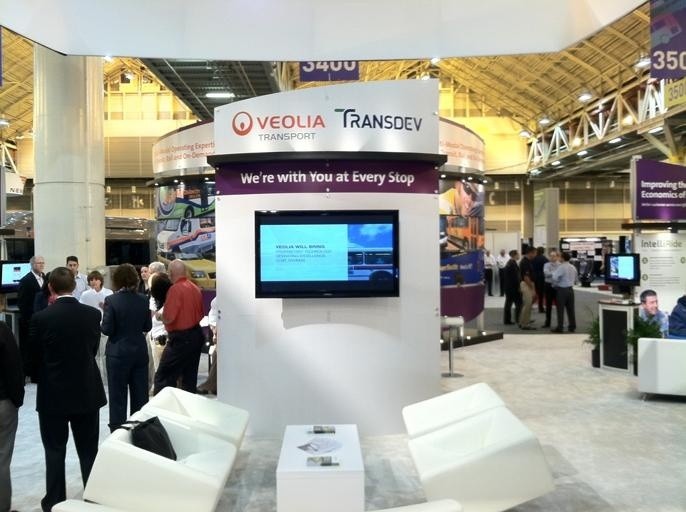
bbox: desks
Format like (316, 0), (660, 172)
(568, 285), (623, 329)
(273, 421), (366, 511)
(596, 295), (639, 376)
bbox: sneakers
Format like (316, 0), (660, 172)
(195, 386), (209, 395)
(486, 292), (577, 334)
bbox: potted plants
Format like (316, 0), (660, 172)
(623, 317), (662, 376)
(582, 314), (601, 365)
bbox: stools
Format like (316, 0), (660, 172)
(84, 423), (237, 511)
(440, 315), (465, 379)
(402, 380), (508, 434)
(400, 406), (557, 512)
(143, 381), (253, 452)
(637, 338), (685, 399)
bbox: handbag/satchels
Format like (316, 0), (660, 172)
(108, 415), (178, 461)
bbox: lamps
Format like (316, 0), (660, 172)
(518, 129), (533, 139)
(636, 48), (650, 71)
(579, 83), (592, 104)
(540, 112), (554, 127)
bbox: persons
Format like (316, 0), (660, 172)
(484, 247), (578, 332)
(99, 263), (152, 433)
(0, 256), (173, 396)
(640, 290), (669, 338)
(26, 267), (107, 512)
(669, 295), (686, 337)
(0, 320), (25, 512)
(153, 259), (204, 395)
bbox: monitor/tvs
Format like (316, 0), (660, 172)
(105, 239), (150, 267)
(1, 263), (30, 286)
(605, 254), (641, 286)
(4, 238), (33, 259)
(254, 209), (400, 296)
